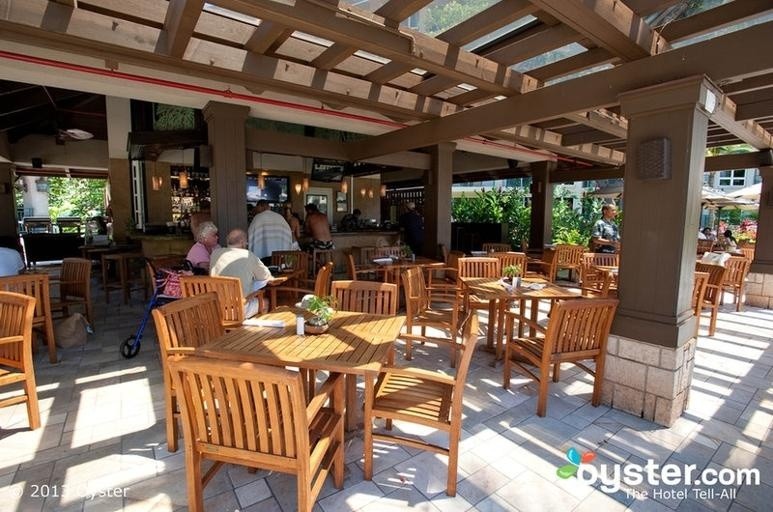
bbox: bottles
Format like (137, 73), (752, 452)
(169, 169), (210, 229)
(411, 254), (415, 261)
(296, 315), (304, 335)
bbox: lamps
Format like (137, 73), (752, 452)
(302, 156), (310, 195)
(257, 150), (263, 189)
(179, 143), (186, 190)
(151, 156), (159, 191)
(293, 174), (302, 196)
(341, 173), (387, 201)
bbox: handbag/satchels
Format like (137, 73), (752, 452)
(171, 259), (207, 294)
(52, 312), (93, 350)
(154, 266), (192, 298)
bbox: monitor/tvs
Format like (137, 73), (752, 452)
(129, 99), (208, 132)
(311, 163), (344, 183)
(245, 175), (291, 204)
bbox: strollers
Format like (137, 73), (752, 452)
(119, 257), (208, 360)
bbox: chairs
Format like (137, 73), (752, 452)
(0, 215), (761, 511)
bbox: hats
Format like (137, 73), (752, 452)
(281, 200), (294, 210)
(405, 201), (416, 211)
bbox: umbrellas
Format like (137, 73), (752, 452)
(591, 181), (623, 196)
(700, 183), (763, 239)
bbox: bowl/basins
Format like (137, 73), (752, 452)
(373, 258), (394, 265)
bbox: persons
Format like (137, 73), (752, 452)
(724, 230), (737, 253)
(393, 203), (423, 253)
(339, 208), (363, 232)
(591, 202), (621, 257)
(185, 198), (333, 319)
